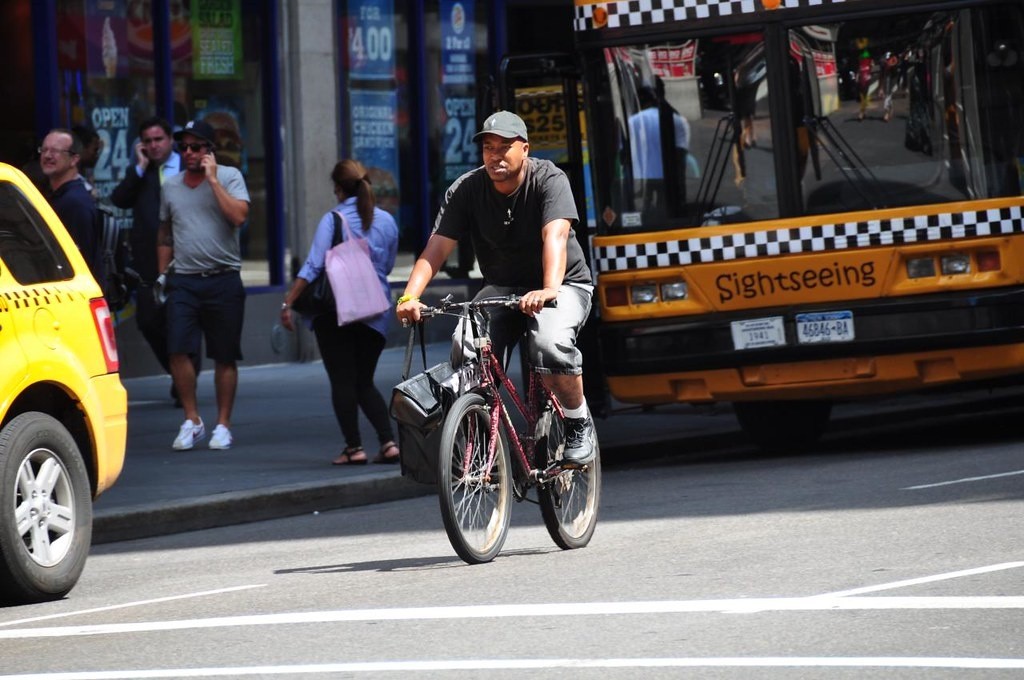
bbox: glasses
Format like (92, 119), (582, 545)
(37, 146), (75, 155)
(143, 136), (163, 144)
(177, 142), (209, 152)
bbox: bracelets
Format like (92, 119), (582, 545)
(397, 294), (420, 303)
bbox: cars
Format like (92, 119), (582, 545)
(1, 159), (127, 600)
(402, 293), (606, 568)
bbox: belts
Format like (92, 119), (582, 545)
(195, 266), (232, 276)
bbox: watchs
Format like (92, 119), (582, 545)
(281, 301), (290, 310)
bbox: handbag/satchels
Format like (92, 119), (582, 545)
(390, 302), (469, 484)
(325, 209), (392, 327)
(292, 213), (343, 316)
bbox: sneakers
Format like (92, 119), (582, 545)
(208, 424), (233, 449)
(172, 416), (206, 450)
(563, 417), (598, 464)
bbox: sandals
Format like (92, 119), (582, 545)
(332, 448), (368, 465)
(372, 444), (401, 464)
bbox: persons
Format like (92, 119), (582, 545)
(155, 120), (251, 451)
(853, 54), (908, 126)
(618, 74), (693, 214)
(282, 159), (402, 466)
(396, 110), (597, 470)
(110, 114), (202, 409)
(38, 128), (105, 293)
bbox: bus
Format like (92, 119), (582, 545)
(500, 1), (1023, 458)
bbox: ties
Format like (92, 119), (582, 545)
(158, 165), (164, 186)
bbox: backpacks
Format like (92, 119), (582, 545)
(91, 207), (134, 311)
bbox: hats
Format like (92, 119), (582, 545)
(173, 120), (215, 145)
(472, 110), (528, 144)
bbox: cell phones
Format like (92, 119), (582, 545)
(206, 147), (217, 158)
(141, 149), (149, 158)
